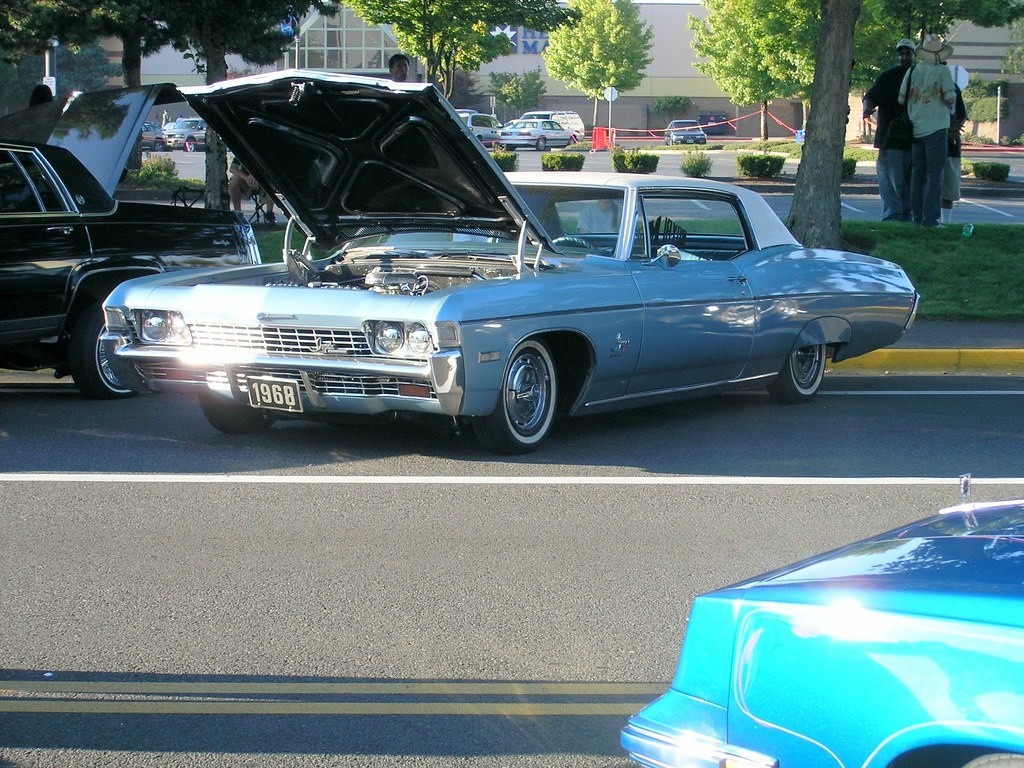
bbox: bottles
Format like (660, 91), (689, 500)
(963, 223), (974, 237)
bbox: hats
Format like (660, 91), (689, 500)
(896, 38), (915, 50)
(915, 34), (953, 64)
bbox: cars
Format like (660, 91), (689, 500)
(96, 68), (920, 452)
(496, 111), (585, 139)
(494, 119), (581, 151)
(409, 108), (504, 148)
(620, 497), (1024, 768)
(161, 118), (230, 153)
(0, 83), (263, 399)
(139, 122), (167, 152)
(664, 120), (706, 146)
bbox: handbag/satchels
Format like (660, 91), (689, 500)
(883, 117), (913, 150)
(948, 135), (961, 158)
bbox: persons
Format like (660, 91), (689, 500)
(576, 199), (622, 233)
(941, 84), (967, 223)
(162, 110), (171, 127)
(177, 115), (183, 122)
(229, 157), (277, 224)
(862, 39), (915, 222)
(898, 34), (955, 228)
(29, 84), (53, 108)
(389, 55), (409, 81)
